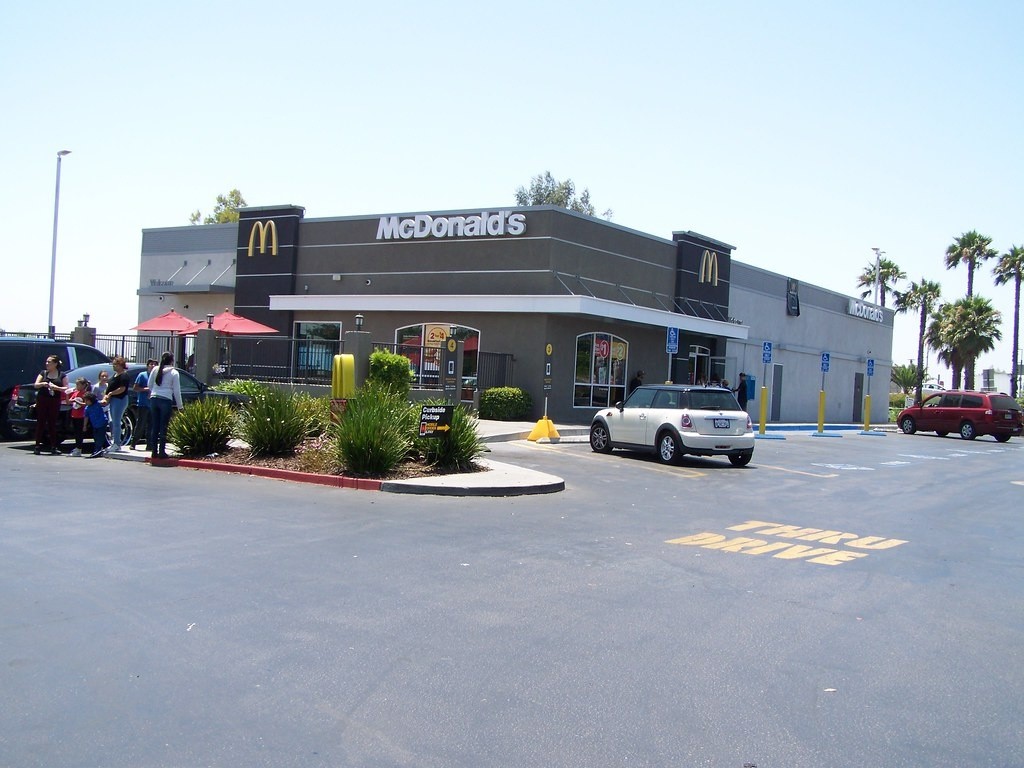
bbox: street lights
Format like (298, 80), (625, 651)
(45, 148), (73, 340)
(872, 247), (885, 305)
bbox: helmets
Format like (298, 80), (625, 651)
(637, 369), (647, 376)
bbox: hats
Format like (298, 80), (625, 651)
(722, 380), (729, 385)
(739, 372), (747, 376)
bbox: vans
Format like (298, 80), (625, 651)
(0, 336), (113, 442)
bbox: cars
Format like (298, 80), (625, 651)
(6, 361), (259, 448)
(911, 382), (948, 396)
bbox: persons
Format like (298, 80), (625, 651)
(698, 372), (747, 413)
(630, 370), (644, 394)
(35, 349), (195, 458)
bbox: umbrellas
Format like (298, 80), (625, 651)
(130, 305), (279, 381)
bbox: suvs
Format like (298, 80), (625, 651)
(588, 383), (756, 467)
(897, 389), (1024, 443)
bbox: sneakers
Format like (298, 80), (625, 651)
(146, 446), (158, 451)
(151, 453), (159, 458)
(33, 447), (40, 455)
(90, 448), (105, 457)
(130, 445), (135, 449)
(69, 449), (82, 457)
(51, 446), (61, 454)
(159, 452), (170, 458)
(106, 443), (122, 451)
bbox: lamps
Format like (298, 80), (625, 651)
(78, 318), (83, 327)
(354, 313), (364, 330)
(83, 312), (90, 327)
(450, 324), (458, 336)
(206, 312), (214, 329)
